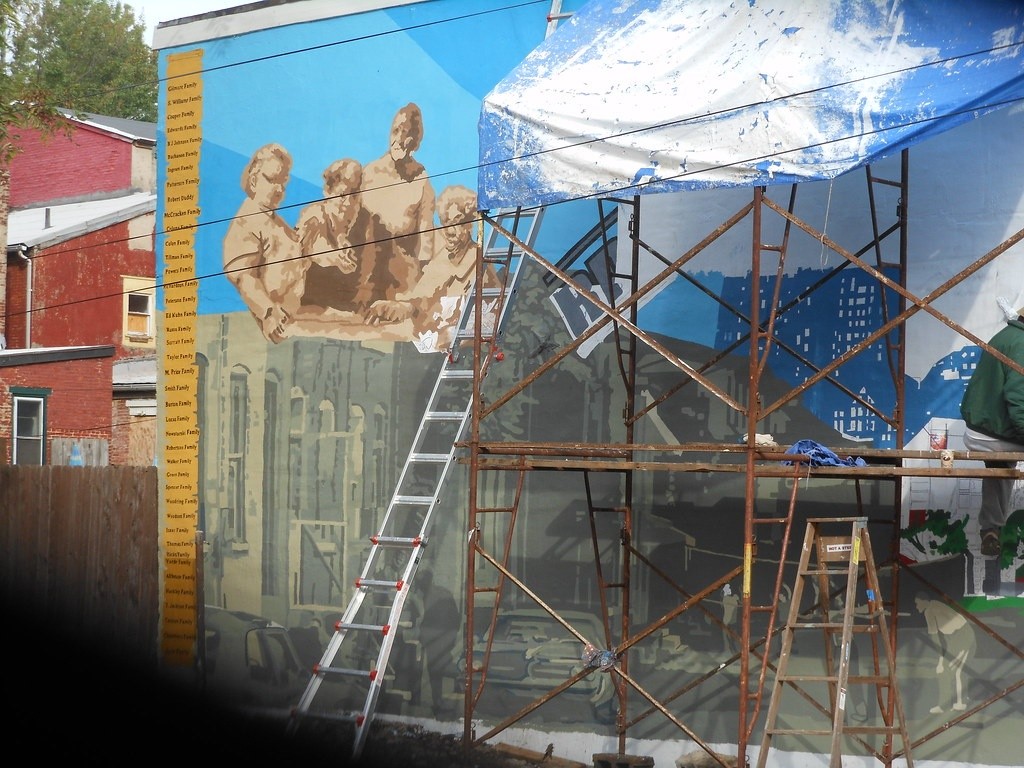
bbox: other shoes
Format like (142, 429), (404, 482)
(981, 533), (1000, 556)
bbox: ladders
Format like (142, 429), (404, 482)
(757, 517), (914, 768)
(271, 202), (545, 768)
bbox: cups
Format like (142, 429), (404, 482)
(930, 419), (948, 451)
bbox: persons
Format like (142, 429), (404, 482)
(955, 307), (1024, 556)
